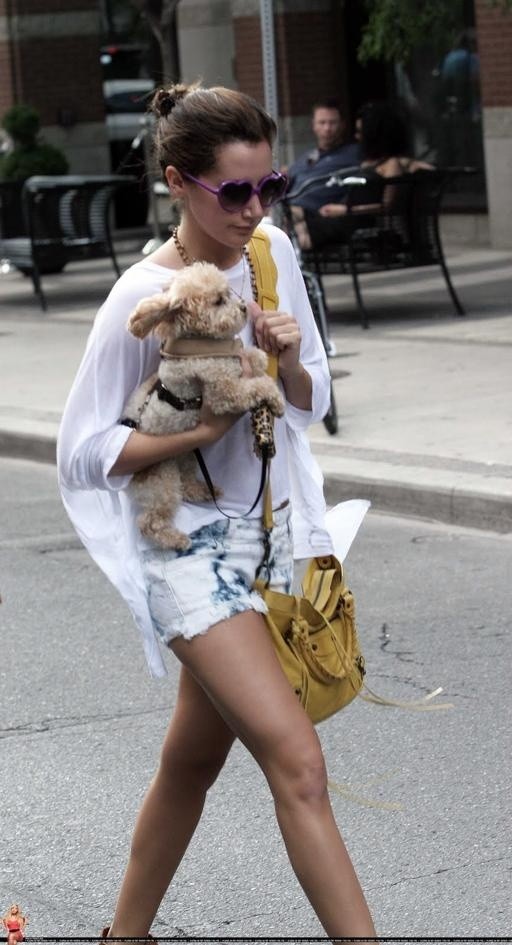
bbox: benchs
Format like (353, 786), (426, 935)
(2, 175), (140, 313)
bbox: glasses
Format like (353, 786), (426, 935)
(184, 170), (289, 214)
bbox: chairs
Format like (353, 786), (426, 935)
(278, 158), (466, 331)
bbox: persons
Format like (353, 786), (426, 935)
(0, 104), (70, 239)
(56, 83), (380, 945)
(280, 102), (438, 264)
(441, 34), (481, 124)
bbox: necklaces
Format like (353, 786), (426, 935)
(173, 225), (258, 303)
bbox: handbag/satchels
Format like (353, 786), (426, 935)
(255, 554), (367, 725)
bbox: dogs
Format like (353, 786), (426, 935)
(117, 259), (288, 550)
